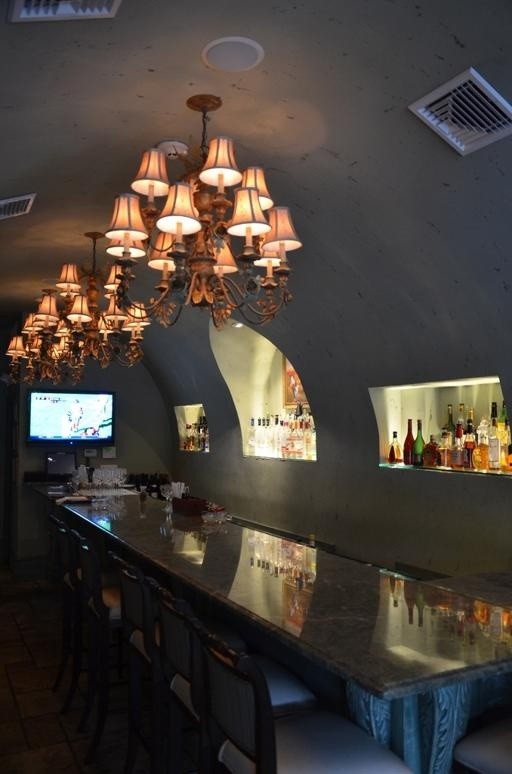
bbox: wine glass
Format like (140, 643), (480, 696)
(93, 468), (127, 491)
(160, 485), (174, 511)
(160, 512), (175, 536)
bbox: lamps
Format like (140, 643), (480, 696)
(106, 94), (303, 331)
(5, 230), (152, 387)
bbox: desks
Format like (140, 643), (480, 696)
(60, 496), (512, 774)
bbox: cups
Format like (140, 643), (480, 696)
(215, 512), (232, 523)
(200, 511), (216, 523)
(171, 482), (189, 499)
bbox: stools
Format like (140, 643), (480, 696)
(452, 718), (512, 774)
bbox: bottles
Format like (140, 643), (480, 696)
(403, 401), (512, 472)
(184, 422), (209, 452)
(248, 529), (317, 591)
(127, 473), (164, 500)
(389, 578), (512, 648)
(388, 432), (403, 464)
(246, 401), (316, 457)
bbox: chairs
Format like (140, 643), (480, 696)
(193, 622), (415, 774)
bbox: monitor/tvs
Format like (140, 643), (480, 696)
(26, 388), (117, 448)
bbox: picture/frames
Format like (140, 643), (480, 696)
(281, 354), (311, 410)
